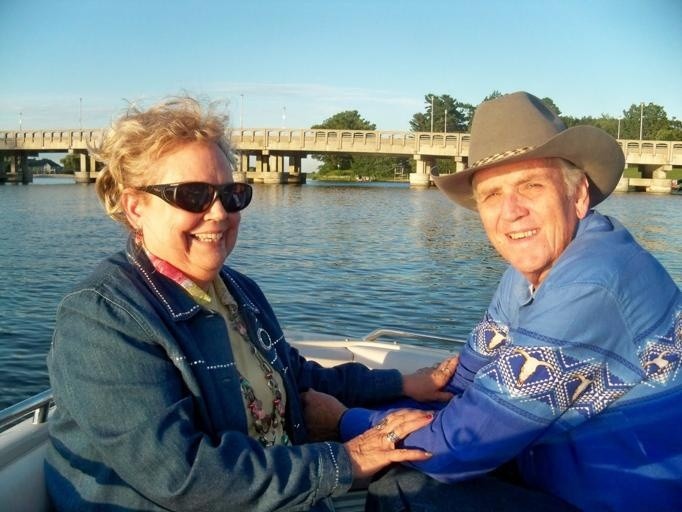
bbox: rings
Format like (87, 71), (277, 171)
(376, 425), (384, 430)
(446, 368), (453, 376)
(388, 433), (401, 442)
(431, 363), (439, 369)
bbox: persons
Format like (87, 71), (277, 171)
(299, 90), (682, 512)
(42, 97), (459, 511)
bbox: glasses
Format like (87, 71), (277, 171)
(127, 181), (252, 212)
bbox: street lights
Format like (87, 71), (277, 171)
(618, 114), (621, 139)
(444, 108), (448, 134)
(281, 105), (287, 129)
(18, 111), (22, 131)
(239, 92), (244, 129)
(78, 95), (83, 128)
(639, 101), (644, 150)
(430, 94), (435, 146)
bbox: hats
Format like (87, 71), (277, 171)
(431, 92), (626, 213)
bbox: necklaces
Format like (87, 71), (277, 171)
(227, 305), (291, 449)
